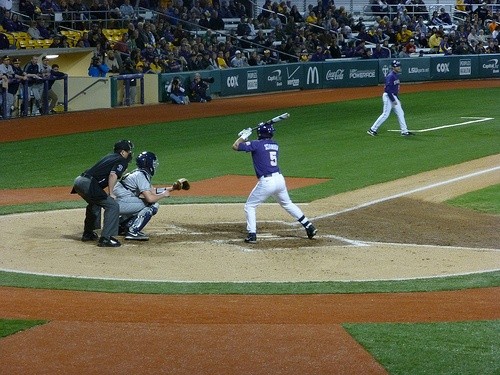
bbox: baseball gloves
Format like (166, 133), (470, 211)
(173, 177), (191, 191)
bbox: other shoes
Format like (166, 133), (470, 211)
(367, 129), (377, 137)
(401, 132), (416, 136)
(125, 231), (149, 240)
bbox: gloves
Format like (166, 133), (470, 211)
(241, 129), (253, 140)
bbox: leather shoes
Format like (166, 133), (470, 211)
(98, 236), (121, 247)
(82, 231), (98, 242)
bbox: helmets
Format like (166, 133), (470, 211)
(256, 122), (275, 139)
(136, 151), (159, 176)
(391, 60), (401, 66)
(114, 140), (134, 163)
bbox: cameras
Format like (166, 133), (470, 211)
(199, 78), (214, 83)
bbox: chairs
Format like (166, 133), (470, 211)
(0, 25), (129, 52)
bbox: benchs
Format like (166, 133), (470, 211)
(324, 20), (458, 61)
(191, 18), (288, 52)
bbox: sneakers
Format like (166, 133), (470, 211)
(306, 226), (317, 239)
(244, 233), (257, 244)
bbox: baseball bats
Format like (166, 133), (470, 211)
(237, 112), (291, 136)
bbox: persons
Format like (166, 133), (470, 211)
(233, 122), (318, 244)
(113, 152), (181, 241)
(0, 0), (500, 119)
(367, 60), (415, 136)
(73, 140), (133, 248)
